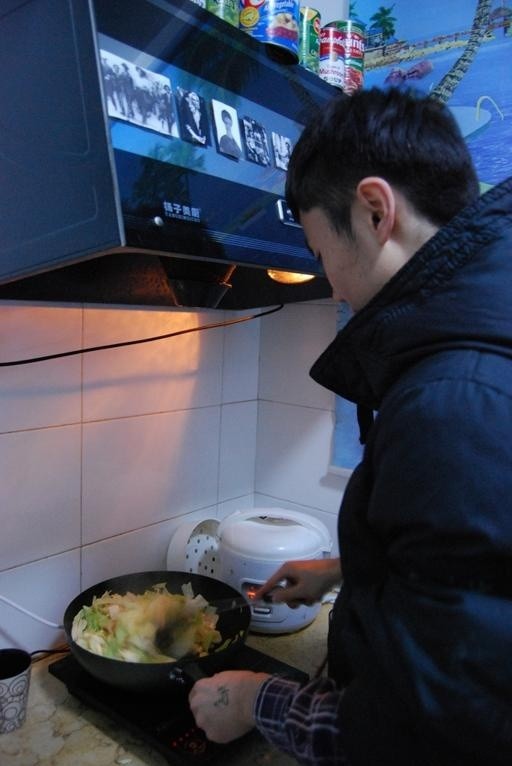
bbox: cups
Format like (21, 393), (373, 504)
(1, 649), (30, 731)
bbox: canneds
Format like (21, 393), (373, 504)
(194, 0), (368, 96)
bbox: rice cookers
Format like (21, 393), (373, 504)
(215, 506), (334, 635)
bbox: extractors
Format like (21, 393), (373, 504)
(1, 0), (354, 310)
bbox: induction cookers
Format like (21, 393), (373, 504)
(48, 642), (309, 759)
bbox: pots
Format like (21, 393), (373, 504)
(61, 569), (251, 690)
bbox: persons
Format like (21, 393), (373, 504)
(275, 140), (292, 169)
(247, 122), (270, 166)
(180, 89), (211, 146)
(187, 85), (509, 766)
(103, 56), (179, 140)
(216, 105), (244, 158)
